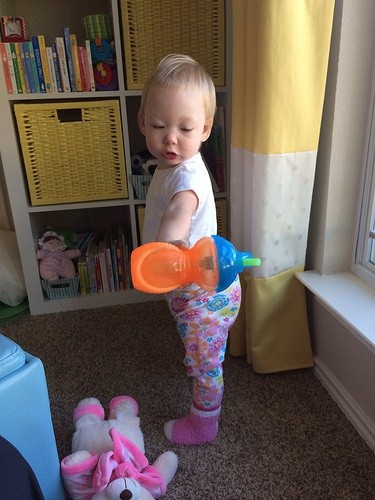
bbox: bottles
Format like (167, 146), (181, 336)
(131, 234), (263, 293)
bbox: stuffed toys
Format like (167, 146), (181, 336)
(61, 394), (180, 500)
(35, 230), (81, 281)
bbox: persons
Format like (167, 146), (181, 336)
(137, 54), (241, 444)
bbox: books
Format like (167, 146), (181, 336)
(0, 27), (96, 94)
(75, 227), (133, 294)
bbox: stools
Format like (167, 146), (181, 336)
(1, 334), (65, 499)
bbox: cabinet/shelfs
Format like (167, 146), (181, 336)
(0, 0), (234, 315)
